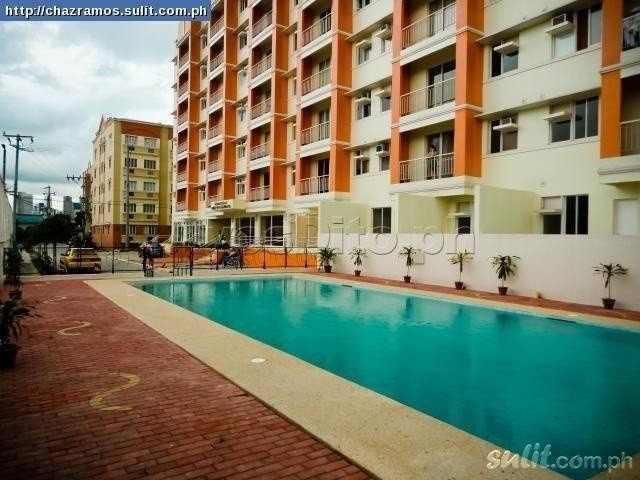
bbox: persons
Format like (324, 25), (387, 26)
(221, 236), (227, 245)
(219, 248), (237, 268)
(44, 251), (53, 267)
(139, 240), (154, 270)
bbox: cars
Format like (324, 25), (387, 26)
(59, 246), (102, 275)
(137, 240), (166, 258)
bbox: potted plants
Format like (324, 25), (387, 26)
(319, 248), (337, 273)
(0, 294), (36, 370)
(448, 248), (472, 290)
(349, 246), (362, 276)
(400, 246), (416, 283)
(593, 263), (626, 309)
(491, 254), (520, 296)
(3, 248), (22, 300)
